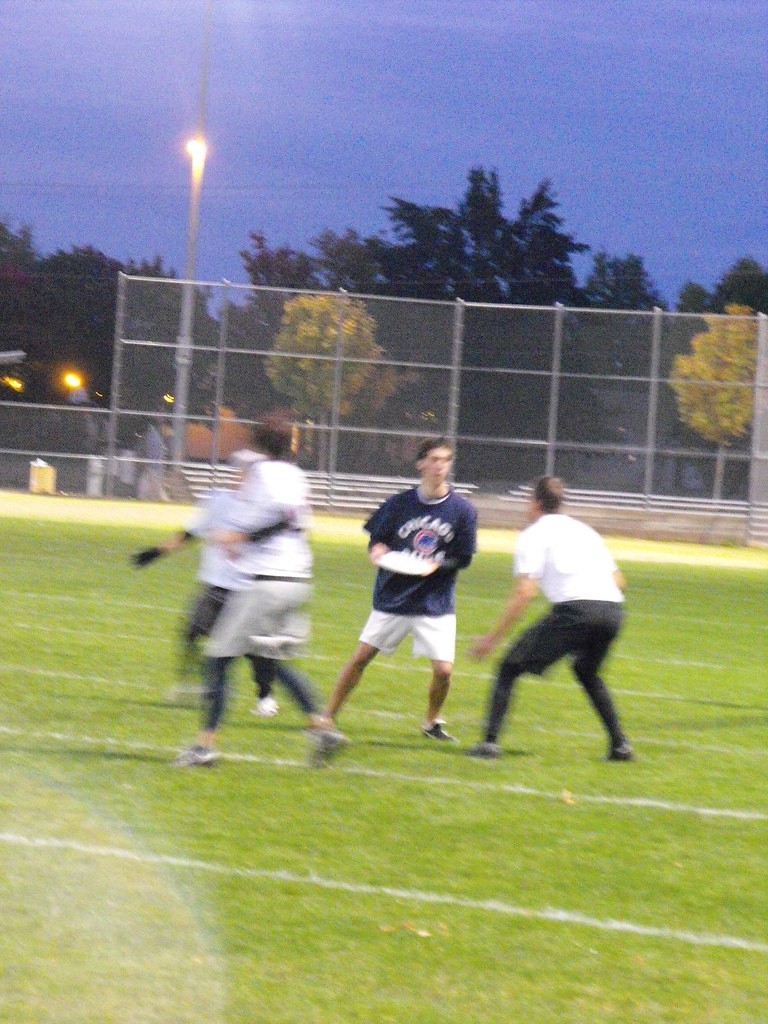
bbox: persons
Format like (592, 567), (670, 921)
(467, 475), (636, 761)
(138, 421), (171, 502)
(126, 408), (352, 768)
(320, 435), (477, 740)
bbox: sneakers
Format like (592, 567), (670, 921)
(424, 724), (459, 745)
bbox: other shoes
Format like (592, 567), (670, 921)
(259, 697), (276, 714)
(314, 732), (347, 762)
(605, 746), (630, 762)
(173, 750), (216, 767)
(468, 743), (498, 760)
